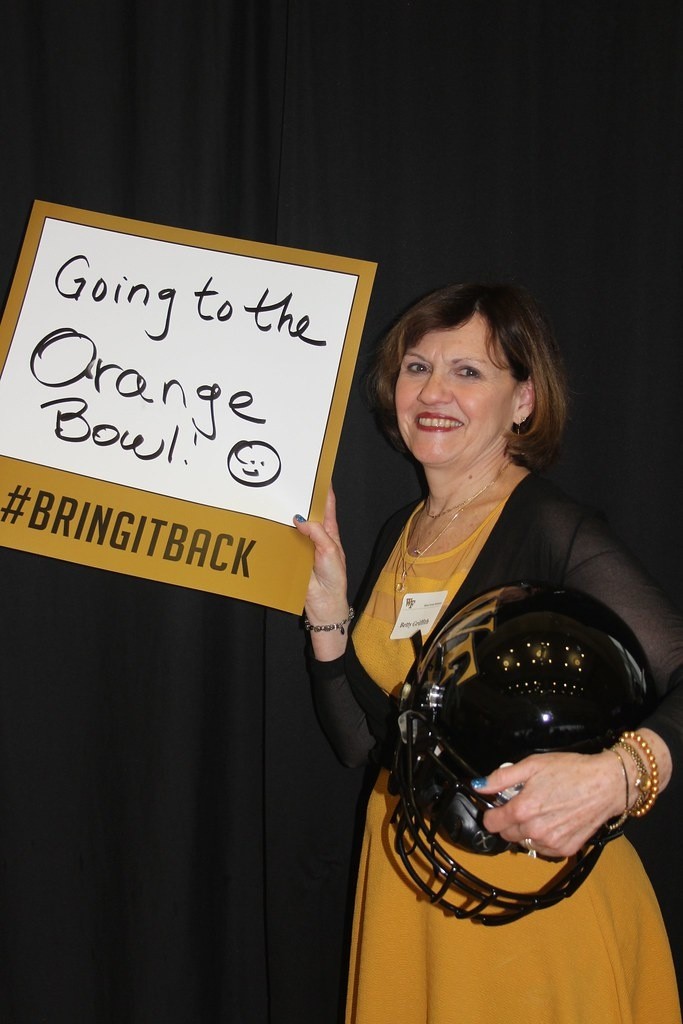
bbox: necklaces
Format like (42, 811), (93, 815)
(395, 460), (514, 592)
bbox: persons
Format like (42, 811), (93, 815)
(299, 285), (683, 1024)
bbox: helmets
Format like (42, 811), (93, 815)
(386, 582), (655, 856)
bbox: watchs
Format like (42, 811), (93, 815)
(612, 741), (651, 813)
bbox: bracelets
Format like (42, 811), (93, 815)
(620, 731), (659, 818)
(602, 747), (629, 830)
(304, 602), (354, 635)
